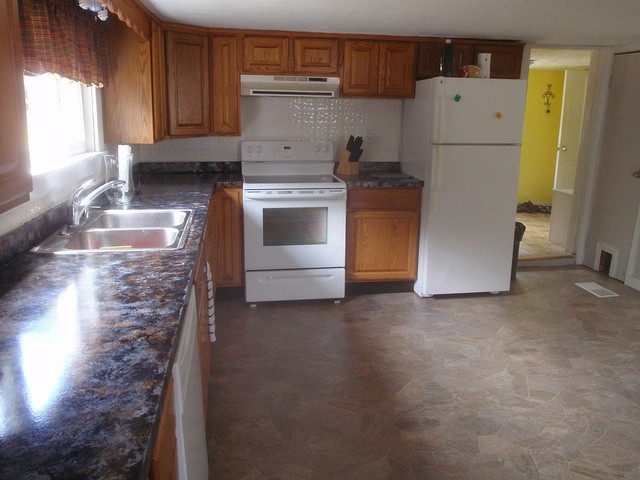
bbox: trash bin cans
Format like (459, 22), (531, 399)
(511, 222), (526, 281)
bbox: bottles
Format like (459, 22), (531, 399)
(441, 39), (454, 77)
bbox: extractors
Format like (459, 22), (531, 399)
(238, 74), (340, 98)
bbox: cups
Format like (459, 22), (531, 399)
(477, 52), (492, 78)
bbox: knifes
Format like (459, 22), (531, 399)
(346, 130), (365, 163)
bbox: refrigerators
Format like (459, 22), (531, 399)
(413, 77), (529, 299)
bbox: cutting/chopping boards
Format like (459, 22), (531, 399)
(370, 169), (412, 180)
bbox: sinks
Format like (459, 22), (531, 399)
(30, 228), (190, 254)
(79, 206), (195, 229)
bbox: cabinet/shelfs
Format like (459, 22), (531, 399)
(0, 1), (33, 214)
(159, 21), (209, 140)
(209, 187), (243, 288)
(151, 375), (179, 480)
(210, 27), (245, 138)
(245, 29), (341, 77)
(196, 283), (213, 426)
(415, 36), (475, 79)
(478, 40), (527, 80)
(100, 17), (166, 146)
(343, 31), (414, 97)
(346, 187), (422, 284)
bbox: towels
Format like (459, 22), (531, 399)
(205, 261), (217, 344)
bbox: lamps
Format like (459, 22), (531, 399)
(541, 82), (555, 114)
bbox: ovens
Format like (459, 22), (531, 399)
(238, 190), (348, 305)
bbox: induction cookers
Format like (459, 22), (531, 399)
(245, 175), (338, 183)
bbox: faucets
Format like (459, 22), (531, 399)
(72, 179), (126, 228)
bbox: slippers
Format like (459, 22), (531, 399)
(537, 205), (551, 214)
